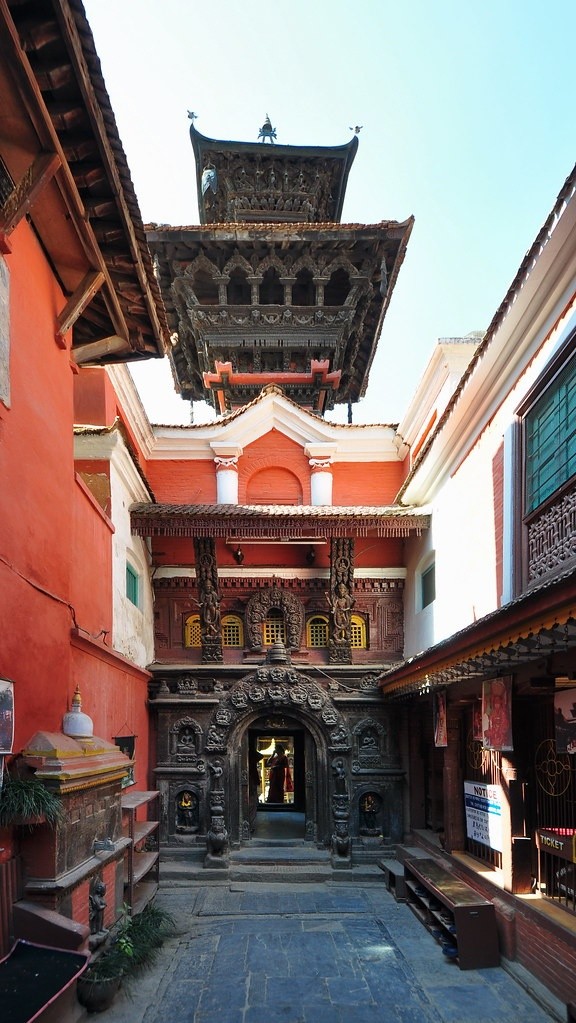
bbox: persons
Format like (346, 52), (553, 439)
(266, 743), (294, 803)
(180, 729), (195, 748)
(362, 731), (378, 749)
(361, 795), (378, 829)
(93, 881), (107, 935)
(179, 794), (195, 826)
(325, 584), (355, 639)
(196, 580), (224, 635)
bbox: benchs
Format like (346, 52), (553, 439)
(375, 857), (410, 904)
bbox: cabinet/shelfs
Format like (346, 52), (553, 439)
(119, 792), (160, 916)
(403, 857), (501, 970)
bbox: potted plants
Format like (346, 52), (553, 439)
(76, 903), (177, 1014)
(0, 772), (64, 825)
